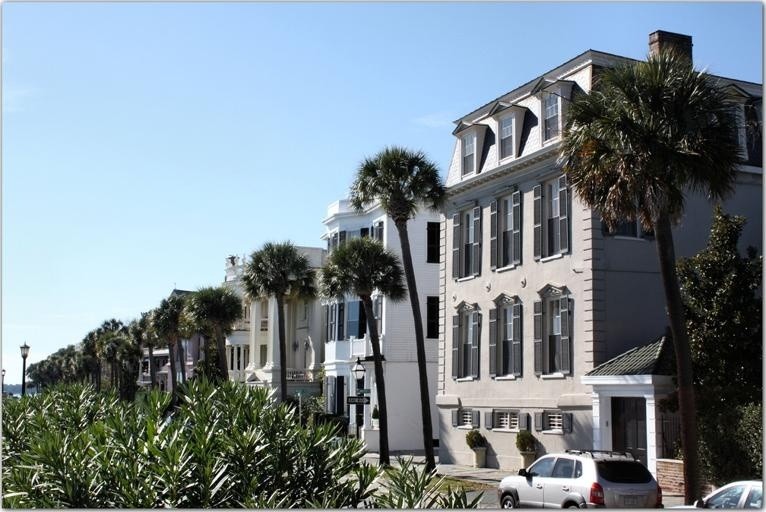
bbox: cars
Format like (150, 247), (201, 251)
(692, 480), (763, 509)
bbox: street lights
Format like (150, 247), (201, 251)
(351, 358), (367, 470)
(1, 369), (5, 392)
(18, 340), (29, 397)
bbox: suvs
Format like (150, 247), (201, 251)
(499, 451), (663, 511)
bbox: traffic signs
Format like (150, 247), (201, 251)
(346, 396), (369, 405)
